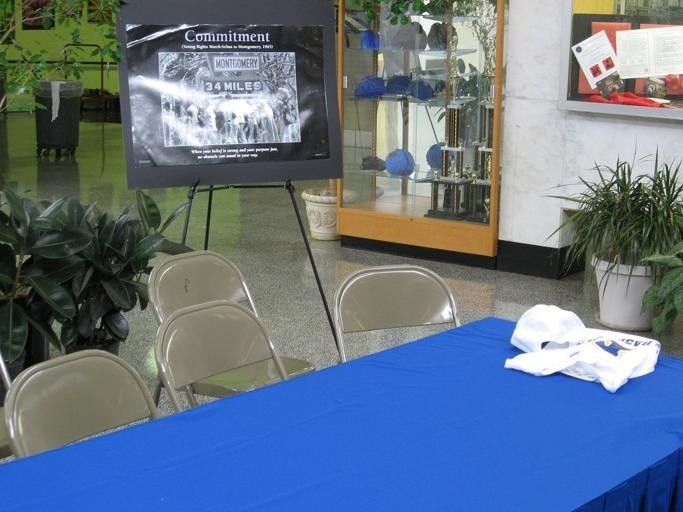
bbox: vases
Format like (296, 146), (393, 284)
(301, 188), (356, 240)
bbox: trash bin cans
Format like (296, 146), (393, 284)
(34, 80), (82, 158)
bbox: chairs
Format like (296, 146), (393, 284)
(5, 349), (158, 461)
(154, 299), (288, 412)
(0, 354), (13, 457)
(148, 250), (315, 398)
(333, 264), (461, 363)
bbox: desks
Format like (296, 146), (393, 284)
(0, 317), (683, 512)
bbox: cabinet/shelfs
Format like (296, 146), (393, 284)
(340, 0), (499, 228)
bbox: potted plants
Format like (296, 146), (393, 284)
(547, 138), (683, 335)
